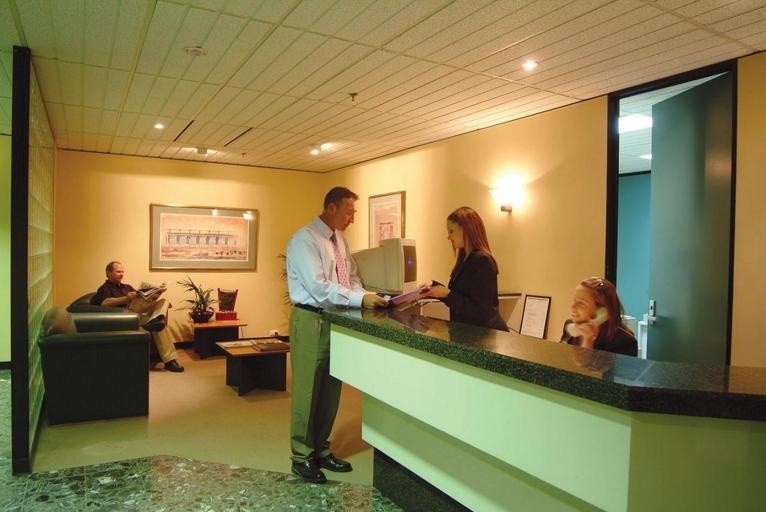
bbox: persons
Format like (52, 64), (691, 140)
(285, 186), (390, 484)
(419, 207), (511, 334)
(558, 275), (638, 358)
(91, 261), (184, 372)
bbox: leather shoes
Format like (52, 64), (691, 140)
(165, 360), (184, 373)
(144, 319), (165, 333)
(314, 454), (352, 472)
(292, 458), (327, 483)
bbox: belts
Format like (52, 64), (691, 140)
(295, 302), (323, 314)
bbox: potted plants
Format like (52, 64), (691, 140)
(175, 275), (219, 322)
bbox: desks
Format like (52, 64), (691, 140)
(188, 320), (247, 357)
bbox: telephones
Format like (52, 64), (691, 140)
(564, 306), (609, 338)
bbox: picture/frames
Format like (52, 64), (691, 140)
(149, 203), (259, 272)
(519, 294), (551, 339)
(368, 190), (406, 248)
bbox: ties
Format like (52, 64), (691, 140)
(330, 233), (349, 289)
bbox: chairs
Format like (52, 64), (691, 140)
(39, 312), (152, 426)
(66, 292), (127, 312)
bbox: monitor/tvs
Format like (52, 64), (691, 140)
(351, 239), (418, 296)
